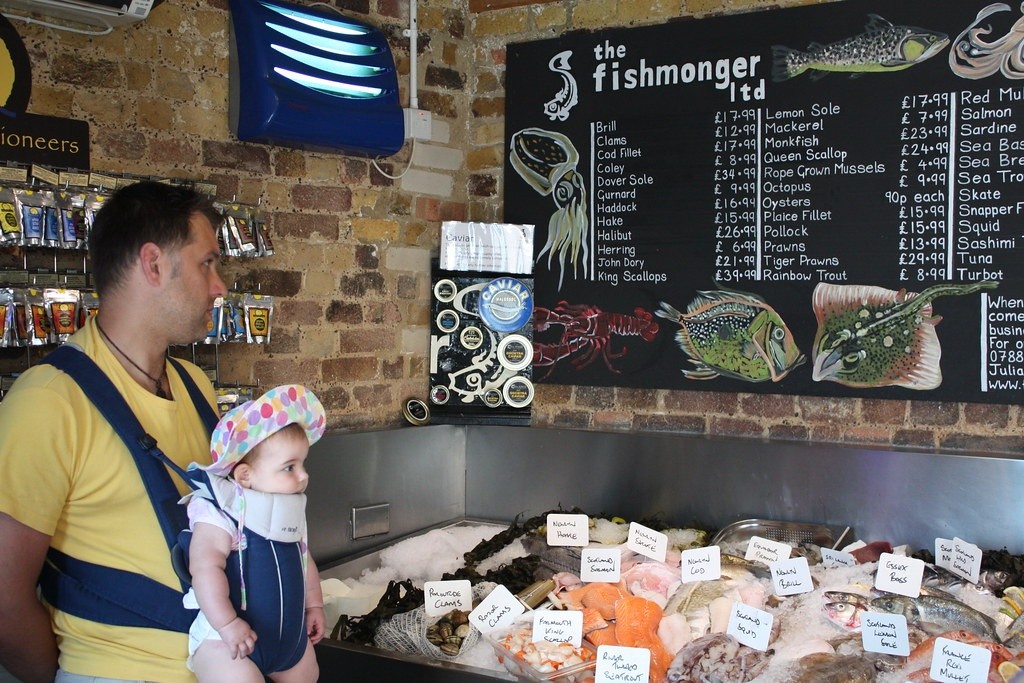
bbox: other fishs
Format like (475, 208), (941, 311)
(487, 518), (1024, 683)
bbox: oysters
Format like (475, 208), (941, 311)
(426, 608), (472, 655)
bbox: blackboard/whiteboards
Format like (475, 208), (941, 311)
(503, 0), (1024, 408)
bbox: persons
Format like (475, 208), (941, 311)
(0, 180), (228, 683)
(177, 384), (327, 683)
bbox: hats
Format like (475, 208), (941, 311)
(185, 385), (327, 478)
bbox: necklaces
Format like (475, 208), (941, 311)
(95, 316), (168, 399)
